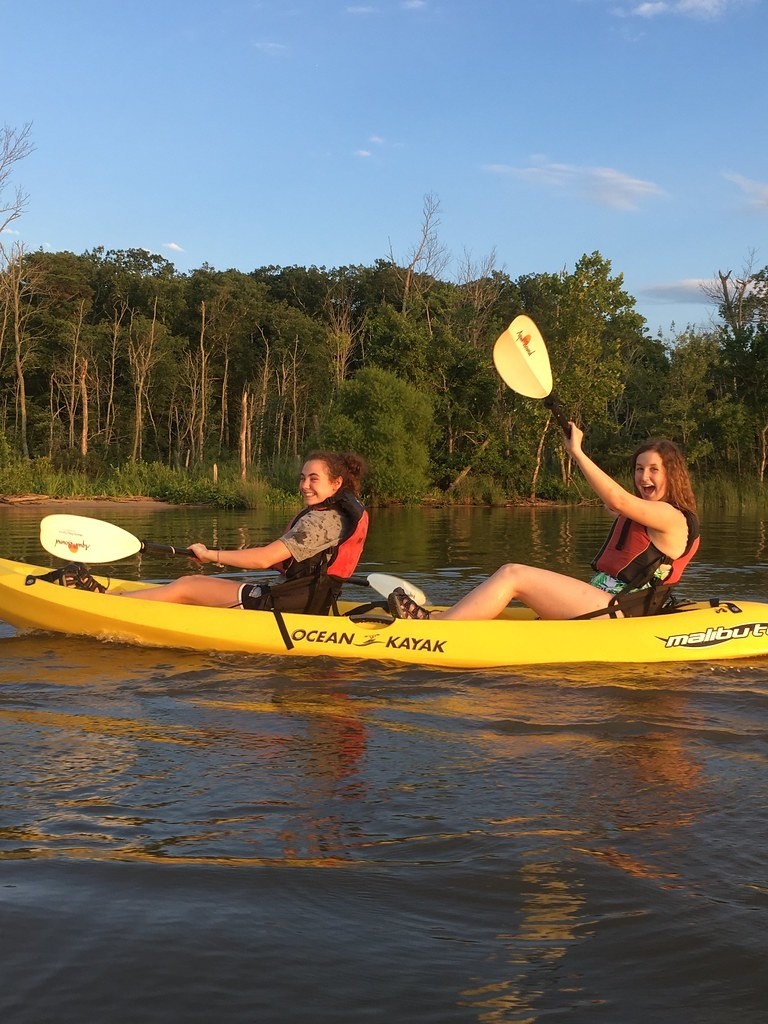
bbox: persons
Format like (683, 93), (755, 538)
(72, 448), (362, 611)
(392, 421), (703, 622)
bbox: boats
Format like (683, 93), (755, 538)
(1, 552), (767, 670)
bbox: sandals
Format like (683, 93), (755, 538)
(388, 587), (431, 620)
(59, 562), (107, 594)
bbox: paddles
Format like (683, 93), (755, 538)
(489, 311), (576, 442)
(37, 512), (429, 609)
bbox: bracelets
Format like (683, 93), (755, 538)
(217, 551), (221, 563)
